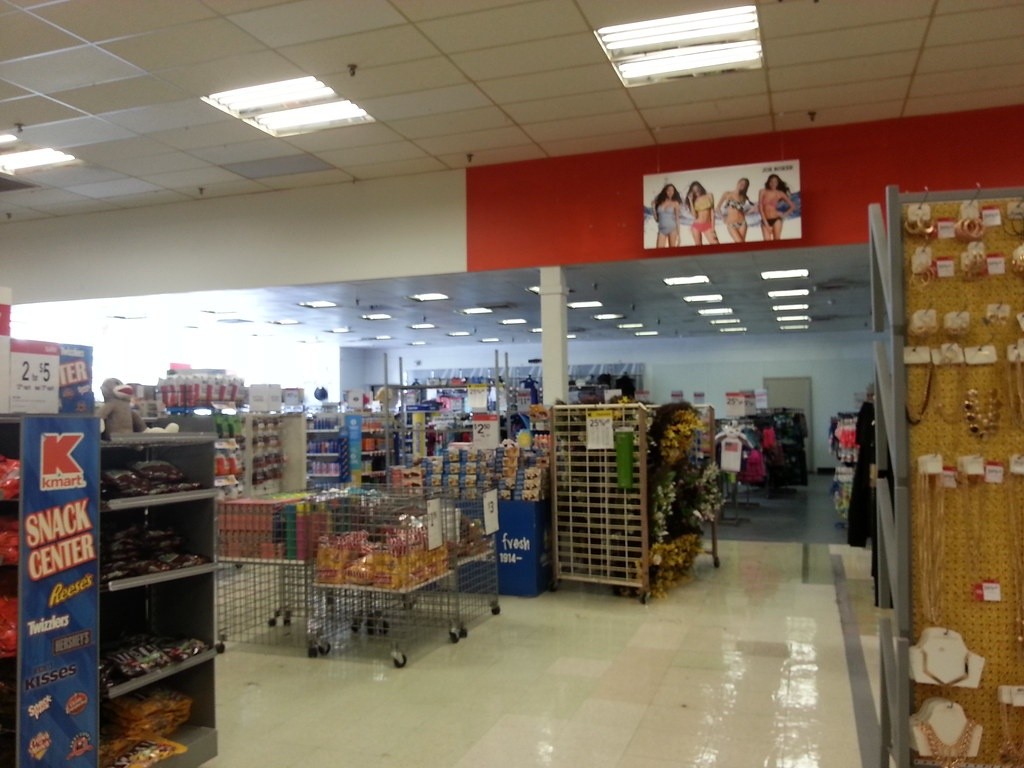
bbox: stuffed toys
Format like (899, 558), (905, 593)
(94, 378), (179, 441)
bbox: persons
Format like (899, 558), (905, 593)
(714, 177), (755, 243)
(650, 183), (682, 248)
(684, 181), (721, 245)
(756, 172), (795, 241)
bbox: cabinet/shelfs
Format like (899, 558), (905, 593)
(0, 337), (718, 768)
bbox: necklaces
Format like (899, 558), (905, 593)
(913, 640), (970, 685)
(914, 710), (978, 768)
(897, 302), (1024, 646)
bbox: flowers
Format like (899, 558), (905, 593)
(636, 408), (726, 600)
